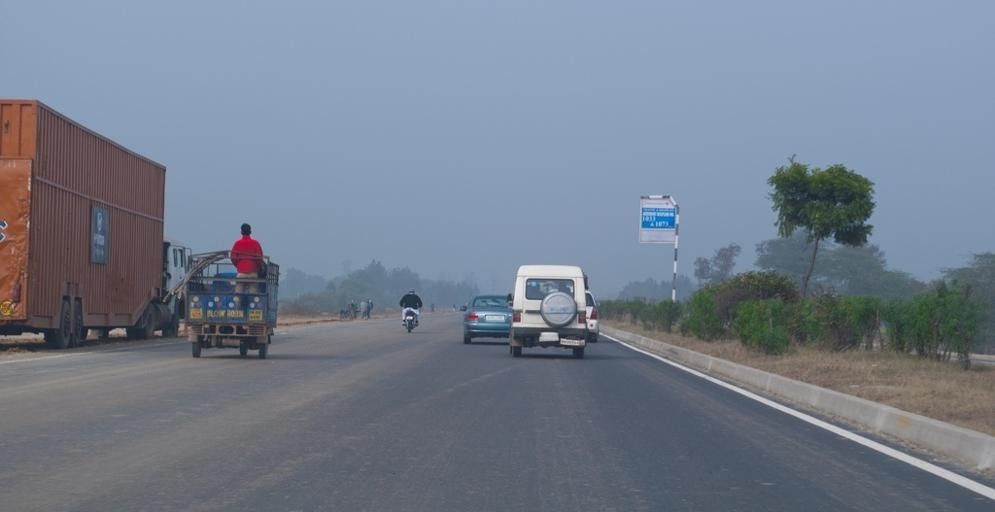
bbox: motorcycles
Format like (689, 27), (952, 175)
(402, 304), (416, 332)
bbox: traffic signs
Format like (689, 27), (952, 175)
(641, 207), (676, 230)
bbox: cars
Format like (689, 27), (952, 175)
(461, 294), (513, 344)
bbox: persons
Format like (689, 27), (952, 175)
(399, 289), (422, 326)
(229, 223), (263, 294)
(349, 297), (373, 319)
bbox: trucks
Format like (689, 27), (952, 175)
(0, 97), (189, 349)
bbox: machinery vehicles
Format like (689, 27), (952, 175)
(187, 256), (279, 360)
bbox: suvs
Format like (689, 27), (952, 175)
(509, 264), (601, 359)
